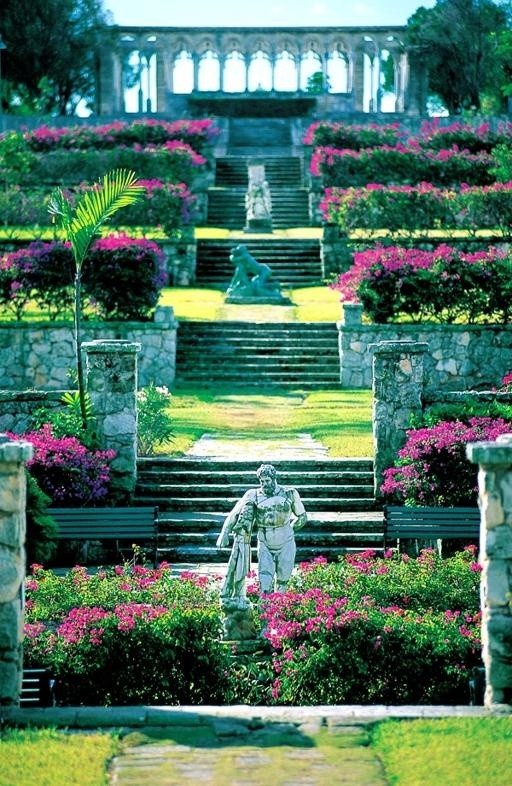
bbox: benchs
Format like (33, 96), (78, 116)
(46, 506), (160, 570)
(382, 505), (479, 560)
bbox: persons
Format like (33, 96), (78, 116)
(216, 463), (308, 595)
(228, 242), (283, 299)
